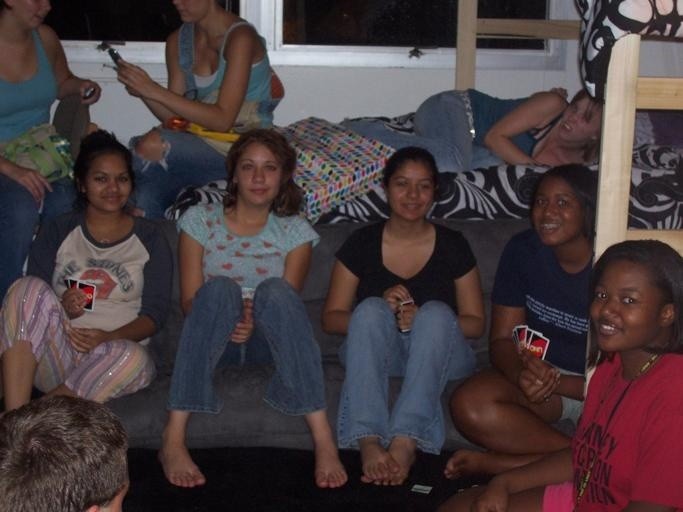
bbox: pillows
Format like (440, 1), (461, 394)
(634, 110), (683, 150)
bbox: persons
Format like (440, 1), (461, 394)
(0, 392), (132, 512)
(443, 160), (597, 480)
(339, 83), (604, 176)
(0, 129), (174, 419)
(0, 0), (100, 304)
(438, 238), (683, 512)
(113, 0), (286, 223)
(155, 128), (349, 488)
(320, 144), (487, 487)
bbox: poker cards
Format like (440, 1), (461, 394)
(63, 277), (96, 313)
(411, 484), (432, 494)
(400, 299), (414, 333)
(512, 323), (550, 362)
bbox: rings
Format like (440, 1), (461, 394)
(544, 395), (551, 405)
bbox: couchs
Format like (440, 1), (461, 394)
(105, 218), (533, 453)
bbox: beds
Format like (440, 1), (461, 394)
(165, 0), (683, 230)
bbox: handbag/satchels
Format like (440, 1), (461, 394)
(1, 122), (77, 185)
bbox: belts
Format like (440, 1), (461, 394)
(460, 88), (474, 136)
